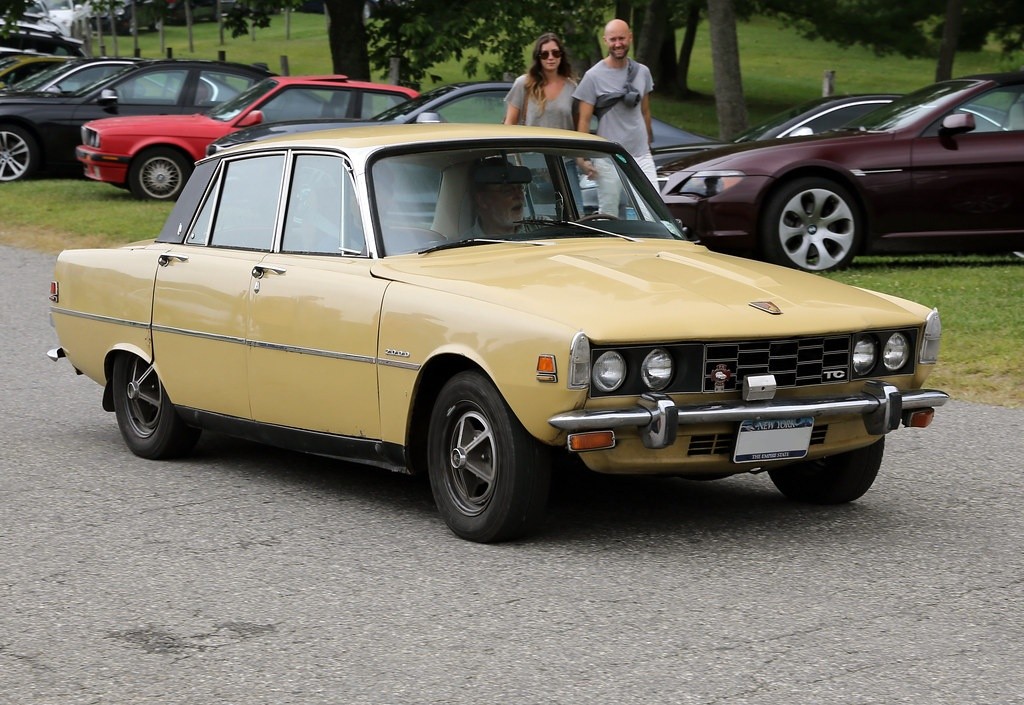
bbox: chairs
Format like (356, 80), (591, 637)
(432, 160), (477, 242)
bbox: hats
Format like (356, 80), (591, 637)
(474, 157), (532, 186)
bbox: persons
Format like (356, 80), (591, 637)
(457, 156), (556, 241)
(570, 19), (661, 223)
(312, 163), (421, 257)
(503, 33), (598, 219)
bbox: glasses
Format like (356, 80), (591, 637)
(480, 185), (527, 197)
(538, 50), (563, 60)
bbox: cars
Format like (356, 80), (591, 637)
(0, 0), (331, 183)
(625, 70), (1024, 273)
(47, 124), (949, 544)
(74, 74), (422, 200)
(207, 78), (723, 226)
(580, 92), (1010, 208)
